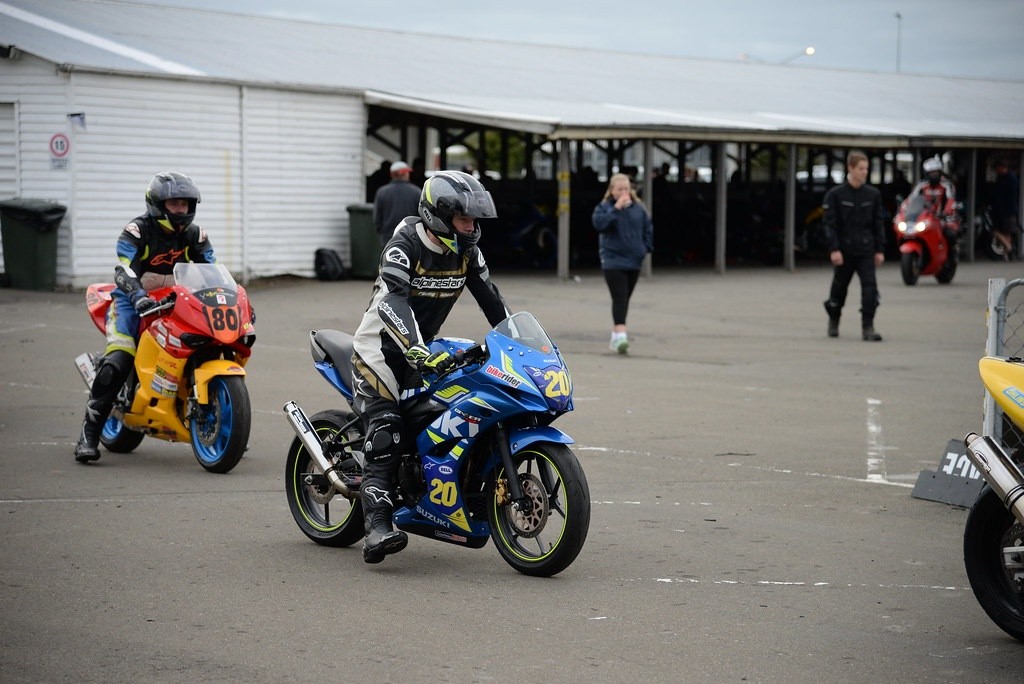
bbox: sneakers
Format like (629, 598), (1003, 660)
(609, 337), (630, 354)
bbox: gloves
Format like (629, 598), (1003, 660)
(135, 297), (158, 317)
(405, 344), (456, 380)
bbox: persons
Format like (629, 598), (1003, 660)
(367, 161), (427, 245)
(899, 157), (959, 263)
(822, 150), (885, 341)
(592, 174), (655, 357)
(351, 171), (521, 564)
(987, 160), (1019, 260)
(75, 172), (215, 468)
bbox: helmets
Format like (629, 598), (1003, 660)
(145, 172), (201, 234)
(923, 158), (943, 179)
(418, 170), (486, 254)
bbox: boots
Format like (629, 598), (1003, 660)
(74, 398), (114, 462)
(359, 478), (408, 564)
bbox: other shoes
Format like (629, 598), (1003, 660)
(828, 319), (839, 337)
(863, 329), (882, 341)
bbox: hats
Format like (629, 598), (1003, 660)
(391, 162), (411, 176)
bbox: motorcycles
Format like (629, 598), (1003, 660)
(964, 353), (1024, 650)
(891, 184), (961, 287)
(485, 183), (1024, 263)
(74, 259), (258, 477)
(281, 310), (592, 579)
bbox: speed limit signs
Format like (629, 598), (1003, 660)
(50, 134), (69, 156)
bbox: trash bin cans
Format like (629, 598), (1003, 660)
(0, 197), (67, 291)
(346, 204), (381, 279)
